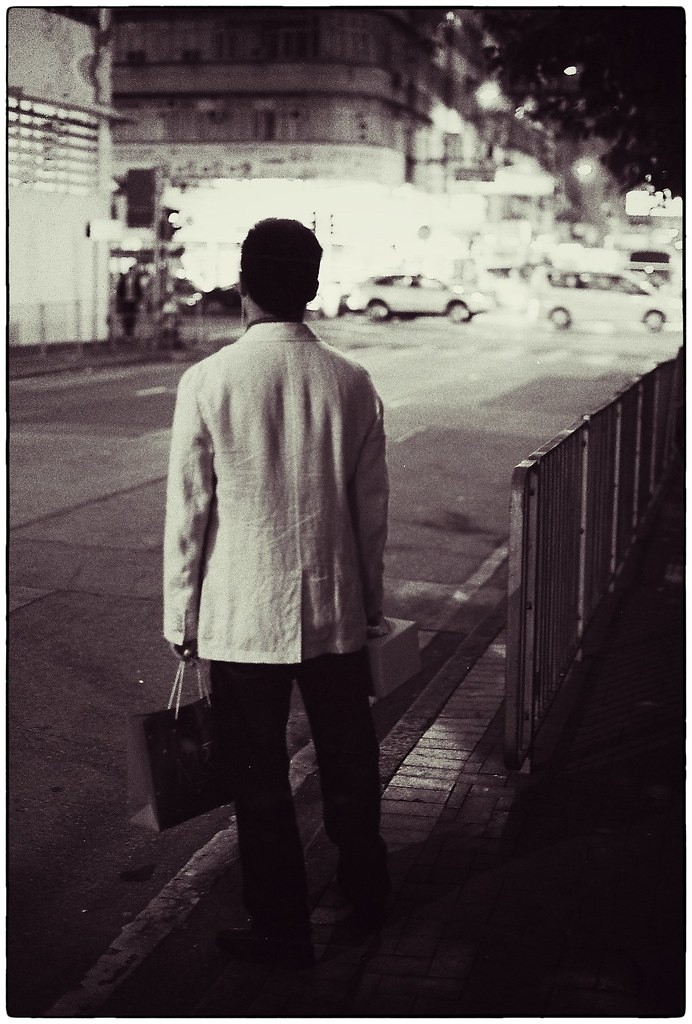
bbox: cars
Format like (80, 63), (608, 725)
(346, 273), (495, 326)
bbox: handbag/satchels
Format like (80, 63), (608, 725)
(127, 653), (237, 833)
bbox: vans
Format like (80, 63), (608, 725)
(540, 269), (685, 334)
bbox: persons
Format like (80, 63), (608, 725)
(115, 268), (143, 336)
(163, 217), (386, 962)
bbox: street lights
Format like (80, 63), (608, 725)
(158, 205), (185, 260)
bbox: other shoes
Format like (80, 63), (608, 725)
(216, 914), (315, 956)
(337, 847), (386, 892)
